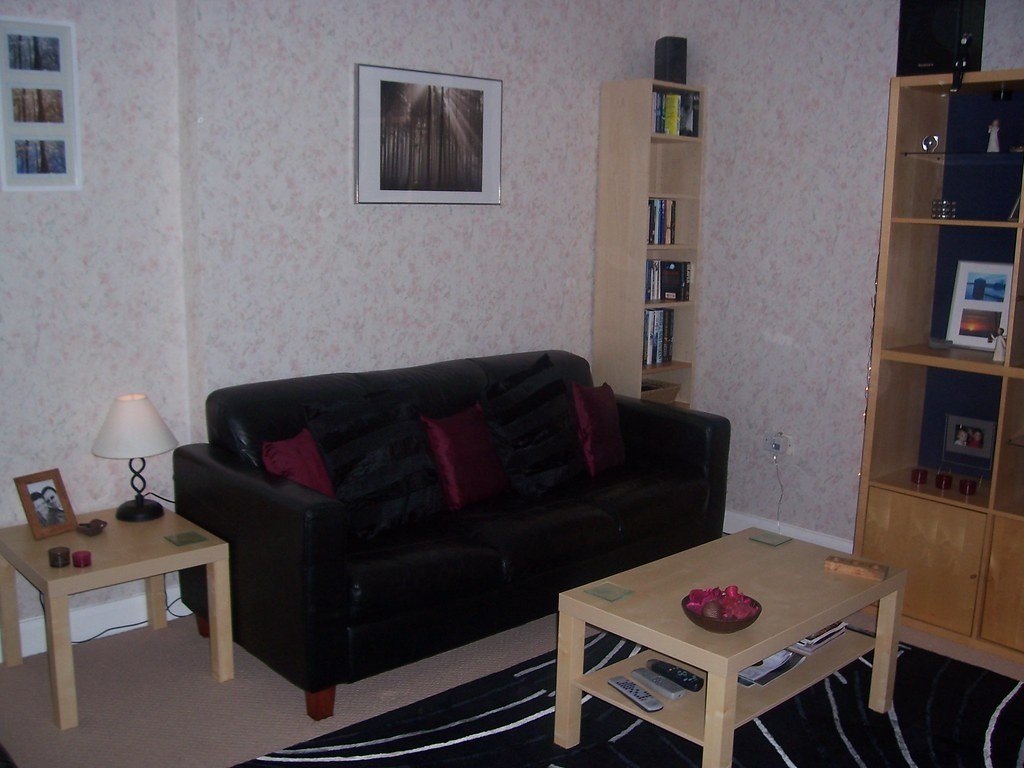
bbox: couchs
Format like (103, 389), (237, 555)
(175, 351), (732, 720)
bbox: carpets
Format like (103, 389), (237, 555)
(230, 630), (1024, 768)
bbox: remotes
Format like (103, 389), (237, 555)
(607, 676), (663, 713)
(648, 659), (704, 692)
(632, 667), (686, 701)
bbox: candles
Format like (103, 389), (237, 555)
(910, 469), (977, 496)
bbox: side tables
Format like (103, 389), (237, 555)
(0, 506), (235, 730)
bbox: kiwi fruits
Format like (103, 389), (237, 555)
(702, 601), (723, 618)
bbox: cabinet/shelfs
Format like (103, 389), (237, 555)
(843, 72), (1024, 681)
(590, 78), (705, 414)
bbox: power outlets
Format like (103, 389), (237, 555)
(778, 433), (793, 456)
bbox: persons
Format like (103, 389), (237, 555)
(991, 328), (1006, 362)
(954, 429), (983, 448)
(31, 486), (65, 527)
(987, 119), (1000, 152)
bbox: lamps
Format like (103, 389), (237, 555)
(93, 395), (179, 522)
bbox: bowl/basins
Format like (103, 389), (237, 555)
(681, 594), (762, 634)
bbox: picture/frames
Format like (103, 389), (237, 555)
(942, 413), (997, 472)
(14, 468), (79, 542)
(354, 63), (504, 207)
(1, 16), (84, 193)
(947, 260), (1013, 351)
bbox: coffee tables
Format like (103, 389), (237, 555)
(555, 527), (909, 768)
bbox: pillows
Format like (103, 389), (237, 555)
(261, 428), (334, 497)
(419, 402), (510, 513)
(567, 380), (626, 477)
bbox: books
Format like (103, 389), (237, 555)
(643, 306), (674, 366)
(652, 91), (700, 136)
(737, 621), (849, 688)
(645, 260), (690, 301)
(649, 199), (676, 245)
